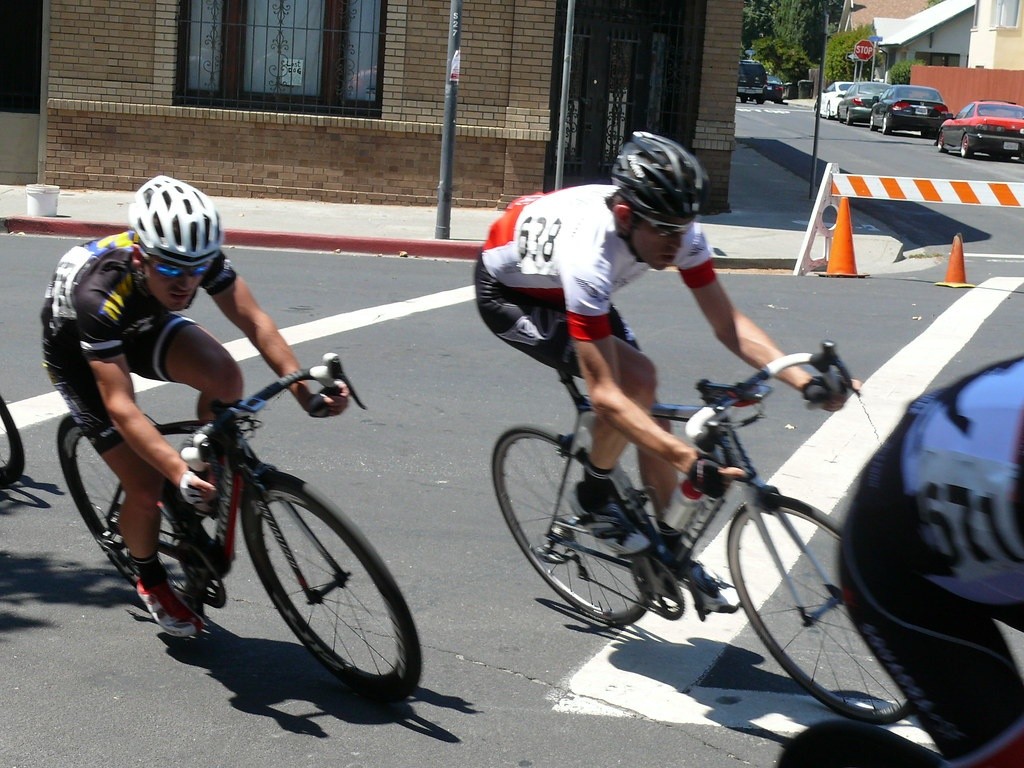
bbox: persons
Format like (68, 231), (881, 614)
(40, 176), (352, 637)
(475, 131), (861, 614)
(840, 354), (1024, 768)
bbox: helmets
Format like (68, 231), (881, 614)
(612, 131), (706, 224)
(128, 176), (224, 265)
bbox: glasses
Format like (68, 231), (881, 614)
(141, 250), (211, 280)
(635, 212), (695, 238)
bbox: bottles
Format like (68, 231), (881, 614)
(179, 442), (207, 487)
(657, 476), (703, 535)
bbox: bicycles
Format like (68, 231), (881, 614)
(0, 394), (25, 488)
(491, 340), (918, 725)
(56, 353), (422, 705)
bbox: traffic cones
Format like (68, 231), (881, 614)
(934, 232), (975, 288)
(815, 197), (870, 278)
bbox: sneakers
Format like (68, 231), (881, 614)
(676, 565), (740, 614)
(136, 579), (205, 637)
(566, 481), (651, 556)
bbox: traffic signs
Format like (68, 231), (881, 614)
(846, 53), (861, 61)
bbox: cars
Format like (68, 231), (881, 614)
(735, 60), (767, 104)
(869, 85), (953, 139)
(937, 99), (1024, 163)
(765, 76), (782, 101)
(837, 82), (891, 126)
(814, 81), (854, 120)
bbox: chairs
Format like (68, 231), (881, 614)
(902, 91), (909, 98)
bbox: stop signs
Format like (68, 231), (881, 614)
(854, 40), (874, 61)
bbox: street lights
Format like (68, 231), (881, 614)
(869, 36), (883, 82)
